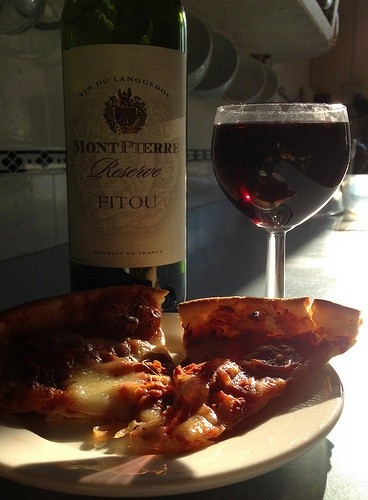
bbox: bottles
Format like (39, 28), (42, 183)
(61, 0), (188, 315)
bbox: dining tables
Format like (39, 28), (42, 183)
(0, 170), (368, 499)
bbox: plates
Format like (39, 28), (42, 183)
(0, 313), (345, 499)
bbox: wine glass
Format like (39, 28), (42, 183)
(212, 102), (350, 301)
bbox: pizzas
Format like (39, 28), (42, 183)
(1, 283), (361, 457)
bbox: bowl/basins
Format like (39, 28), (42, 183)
(194, 31), (239, 98)
(258, 55), (279, 102)
(187, 14), (213, 96)
(226, 54), (266, 102)
